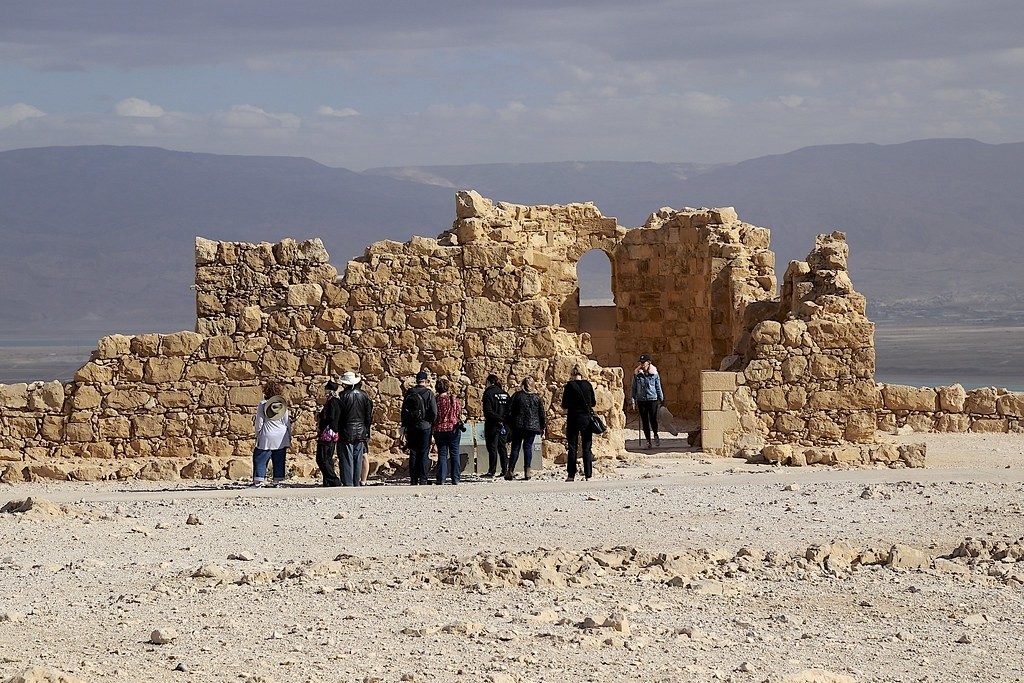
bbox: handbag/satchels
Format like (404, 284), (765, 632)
(589, 410), (606, 434)
(320, 426), (339, 442)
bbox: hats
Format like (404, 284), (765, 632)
(416, 372), (427, 380)
(637, 355), (651, 362)
(339, 371), (361, 386)
(264, 395), (287, 421)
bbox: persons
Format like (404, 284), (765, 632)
(244, 380), (292, 489)
(479, 374), (511, 479)
(629, 354), (665, 450)
(503, 377), (547, 480)
(400, 372), (437, 486)
(562, 364), (597, 482)
(338, 371), (373, 487)
(314, 380), (344, 487)
(431, 378), (464, 486)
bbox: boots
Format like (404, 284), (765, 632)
(504, 468), (514, 480)
(524, 466), (531, 480)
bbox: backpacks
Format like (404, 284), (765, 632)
(401, 388), (432, 423)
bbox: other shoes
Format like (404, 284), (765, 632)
(654, 434), (660, 446)
(645, 438), (652, 448)
(480, 472), (494, 478)
